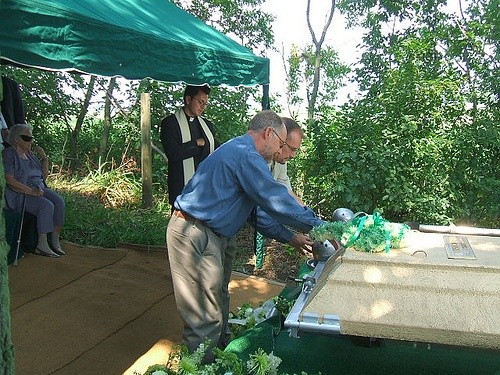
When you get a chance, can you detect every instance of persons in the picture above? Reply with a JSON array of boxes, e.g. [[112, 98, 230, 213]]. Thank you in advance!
[[161, 83, 220, 220], [166, 110, 338, 375], [217, 118, 308, 349], [0, 113, 67, 260]]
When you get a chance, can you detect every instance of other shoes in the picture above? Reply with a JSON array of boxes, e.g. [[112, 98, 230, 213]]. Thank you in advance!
[[35, 248, 59, 257], [51, 245, 65, 255]]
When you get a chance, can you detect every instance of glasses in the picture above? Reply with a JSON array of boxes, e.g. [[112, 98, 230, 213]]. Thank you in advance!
[[286, 142, 298, 153], [19, 134, 33, 141], [273, 130, 286, 148], [193, 97, 210, 107]]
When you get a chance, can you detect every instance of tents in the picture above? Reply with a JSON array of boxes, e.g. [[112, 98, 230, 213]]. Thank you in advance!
[[0, 0, 270, 375]]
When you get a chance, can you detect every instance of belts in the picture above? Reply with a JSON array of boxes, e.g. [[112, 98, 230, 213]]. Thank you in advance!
[[173, 209, 220, 236]]
